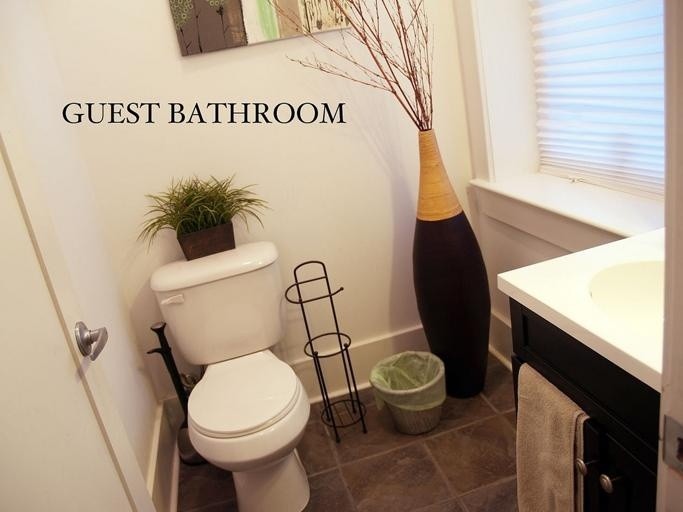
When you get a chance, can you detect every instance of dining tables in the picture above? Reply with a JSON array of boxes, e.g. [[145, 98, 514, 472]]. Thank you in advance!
[[272, 0, 492, 399], [134, 173, 271, 261]]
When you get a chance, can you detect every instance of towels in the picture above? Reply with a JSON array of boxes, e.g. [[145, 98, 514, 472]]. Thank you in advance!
[[369, 351, 445, 435]]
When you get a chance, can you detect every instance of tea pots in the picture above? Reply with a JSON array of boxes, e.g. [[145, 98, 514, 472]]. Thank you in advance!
[[167, 0, 351, 58]]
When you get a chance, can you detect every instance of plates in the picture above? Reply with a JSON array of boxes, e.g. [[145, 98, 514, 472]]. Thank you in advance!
[[498, 226, 664, 510]]
